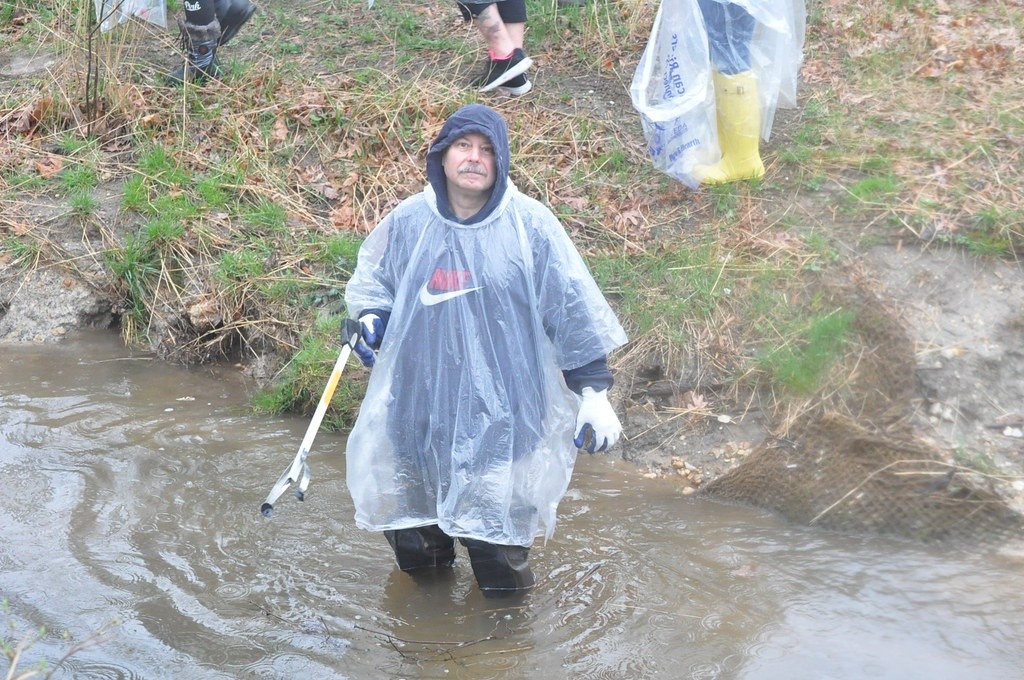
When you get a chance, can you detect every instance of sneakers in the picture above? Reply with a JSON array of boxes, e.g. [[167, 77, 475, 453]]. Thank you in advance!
[[470, 48, 532, 93], [487, 72, 532, 96]]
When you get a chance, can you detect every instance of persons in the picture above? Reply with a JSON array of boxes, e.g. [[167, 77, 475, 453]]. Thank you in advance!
[[691, 0, 766, 186], [456, 0, 534, 96], [350, 104, 623, 594], [162, 0, 257, 87]]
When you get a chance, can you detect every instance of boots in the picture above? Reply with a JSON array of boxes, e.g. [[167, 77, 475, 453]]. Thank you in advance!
[[693, 67, 766, 184], [163, 13, 222, 87], [214, 0, 257, 46]]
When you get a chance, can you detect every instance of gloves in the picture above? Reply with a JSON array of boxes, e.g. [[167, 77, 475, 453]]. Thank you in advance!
[[351, 313, 384, 367], [573, 383, 623, 454]]
